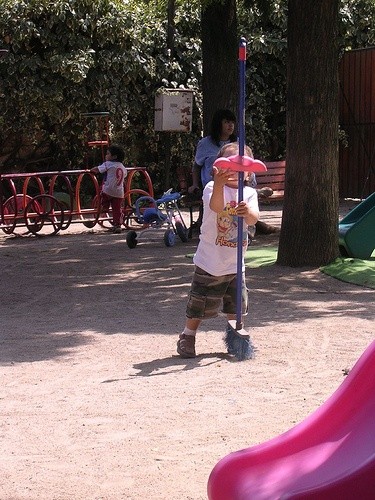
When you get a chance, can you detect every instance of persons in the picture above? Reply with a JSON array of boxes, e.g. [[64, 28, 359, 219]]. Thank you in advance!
[[177, 142, 260, 359], [187, 109, 238, 198], [85, 144, 127, 232]]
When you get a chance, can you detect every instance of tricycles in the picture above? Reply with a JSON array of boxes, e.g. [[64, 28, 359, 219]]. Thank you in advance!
[[126, 193, 189, 248]]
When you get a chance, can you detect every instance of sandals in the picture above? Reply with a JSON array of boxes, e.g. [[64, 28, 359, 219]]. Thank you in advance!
[[228, 341, 258, 353], [177, 333, 196, 358]]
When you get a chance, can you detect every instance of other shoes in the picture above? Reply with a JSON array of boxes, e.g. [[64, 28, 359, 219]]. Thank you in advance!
[[113, 227, 122, 233], [257, 187, 273, 200], [266, 226, 280, 235], [102, 207, 109, 212]]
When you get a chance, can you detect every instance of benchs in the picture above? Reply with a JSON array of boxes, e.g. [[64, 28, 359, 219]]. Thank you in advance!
[[254, 161, 286, 200]]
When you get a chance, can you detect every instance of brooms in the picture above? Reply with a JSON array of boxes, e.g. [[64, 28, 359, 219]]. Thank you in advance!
[[212, 37, 268, 361]]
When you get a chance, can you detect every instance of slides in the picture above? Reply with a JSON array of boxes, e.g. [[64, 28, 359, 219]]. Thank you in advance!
[[207, 337, 375, 500], [338, 190, 375, 259]]
